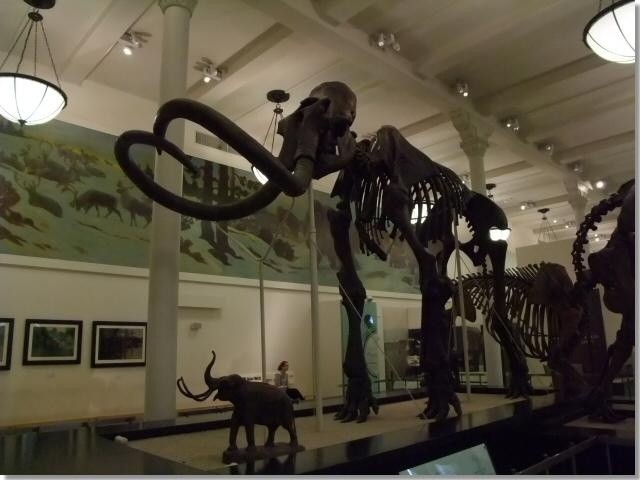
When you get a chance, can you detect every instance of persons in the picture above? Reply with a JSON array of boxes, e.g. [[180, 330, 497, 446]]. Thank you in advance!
[[273, 360, 304, 401]]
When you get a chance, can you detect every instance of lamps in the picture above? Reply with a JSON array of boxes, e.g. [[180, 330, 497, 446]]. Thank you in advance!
[[250, 87, 290, 186], [536, 207, 558, 244], [193, 56, 224, 83], [369, 29, 583, 177], [485, 183, 512, 241], [0, 0, 68, 127], [583, 0, 635, 66], [118, 30, 152, 56]]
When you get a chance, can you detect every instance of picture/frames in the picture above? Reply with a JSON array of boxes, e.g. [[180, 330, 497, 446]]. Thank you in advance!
[[22, 318, 83, 366], [91, 320, 146, 369], [0, 318, 15, 371]]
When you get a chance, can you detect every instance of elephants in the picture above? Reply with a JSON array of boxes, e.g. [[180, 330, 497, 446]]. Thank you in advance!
[[176, 350, 299, 451]]
